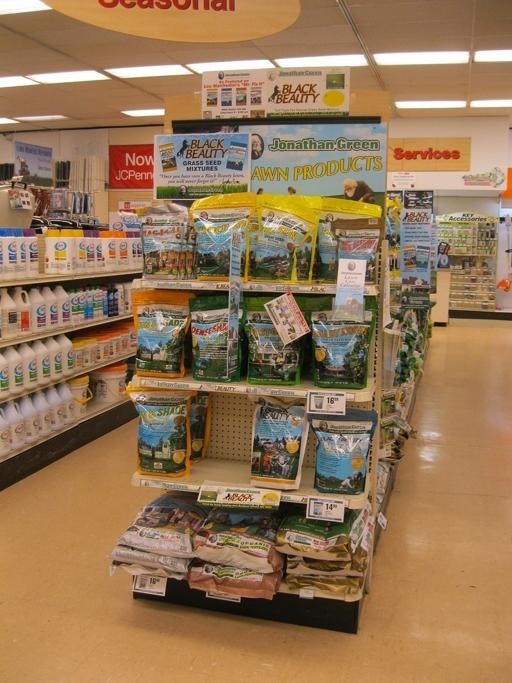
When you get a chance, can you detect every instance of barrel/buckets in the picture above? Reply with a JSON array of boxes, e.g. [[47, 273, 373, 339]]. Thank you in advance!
[[70, 376, 94, 418], [93, 365, 127, 405], [71, 323, 137, 371], [1, 285, 72, 456]]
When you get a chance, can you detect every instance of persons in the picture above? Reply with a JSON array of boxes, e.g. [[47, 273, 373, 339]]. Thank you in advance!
[[343, 179, 374, 203], [251, 136, 262, 159]]
